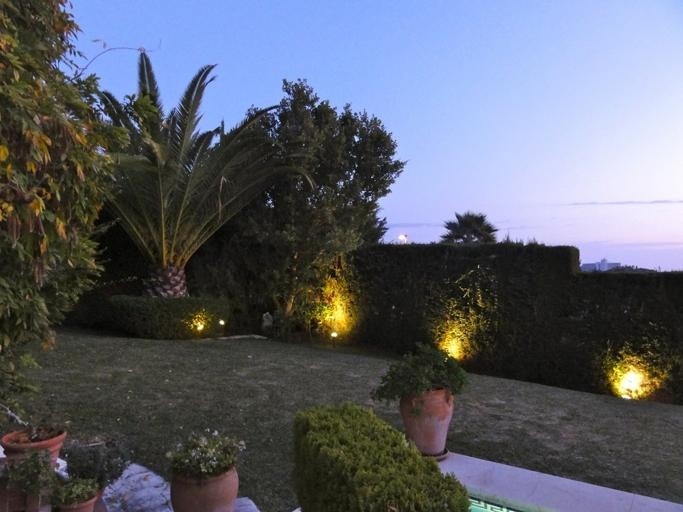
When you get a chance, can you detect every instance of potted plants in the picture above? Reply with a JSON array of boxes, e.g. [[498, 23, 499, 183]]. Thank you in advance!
[[2, 400, 129, 512], [369, 336, 468, 460]]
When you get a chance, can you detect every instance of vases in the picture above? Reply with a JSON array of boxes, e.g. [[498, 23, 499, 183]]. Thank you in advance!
[[170, 469, 241, 512]]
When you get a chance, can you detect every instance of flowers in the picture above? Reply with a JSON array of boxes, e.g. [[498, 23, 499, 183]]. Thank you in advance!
[[161, 424, 249, 483]]
